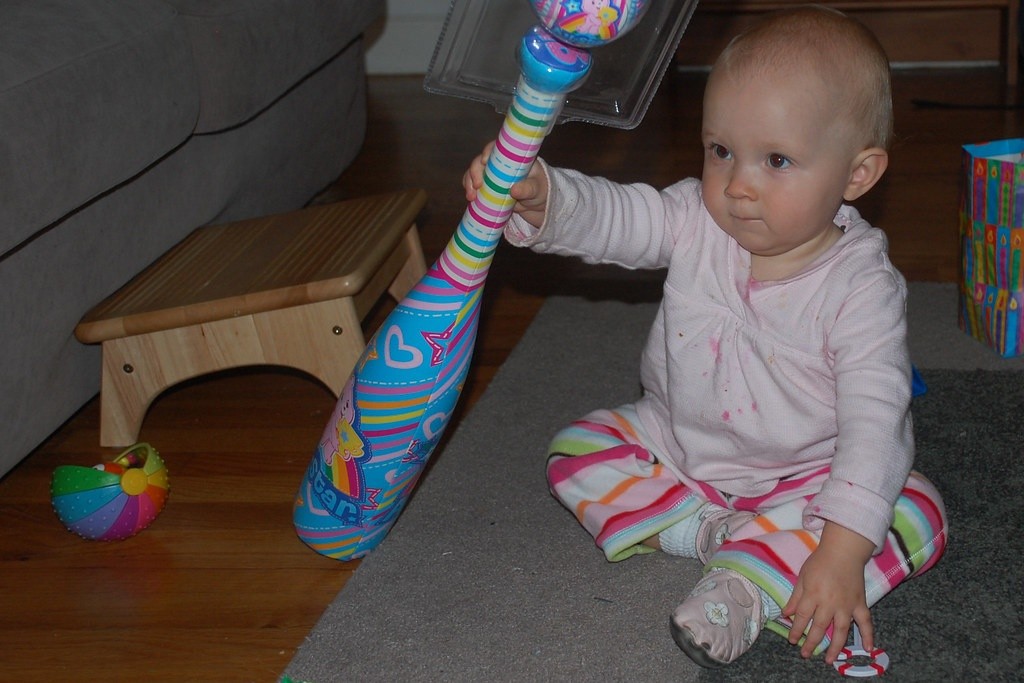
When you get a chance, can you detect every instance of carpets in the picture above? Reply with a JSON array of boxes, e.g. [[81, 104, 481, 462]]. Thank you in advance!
[[280, 280, 1024, 683]]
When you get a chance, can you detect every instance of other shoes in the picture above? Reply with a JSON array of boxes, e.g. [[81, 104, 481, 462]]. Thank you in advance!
[[669, 569, 763, 669], [694, 510, 759, 566]]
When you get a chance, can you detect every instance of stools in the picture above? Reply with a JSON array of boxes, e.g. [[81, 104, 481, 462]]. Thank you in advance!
[[74, 186, 429, 447]]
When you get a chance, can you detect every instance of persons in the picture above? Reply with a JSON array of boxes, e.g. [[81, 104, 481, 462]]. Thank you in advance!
[[461, 6, 949, 669]]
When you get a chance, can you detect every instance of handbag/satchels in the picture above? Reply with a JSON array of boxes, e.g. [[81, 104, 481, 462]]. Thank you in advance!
[[958, 138, 1024, 357]]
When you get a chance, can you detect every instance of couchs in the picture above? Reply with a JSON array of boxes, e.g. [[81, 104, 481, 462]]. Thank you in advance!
[[0, 0, 386, 482]]
